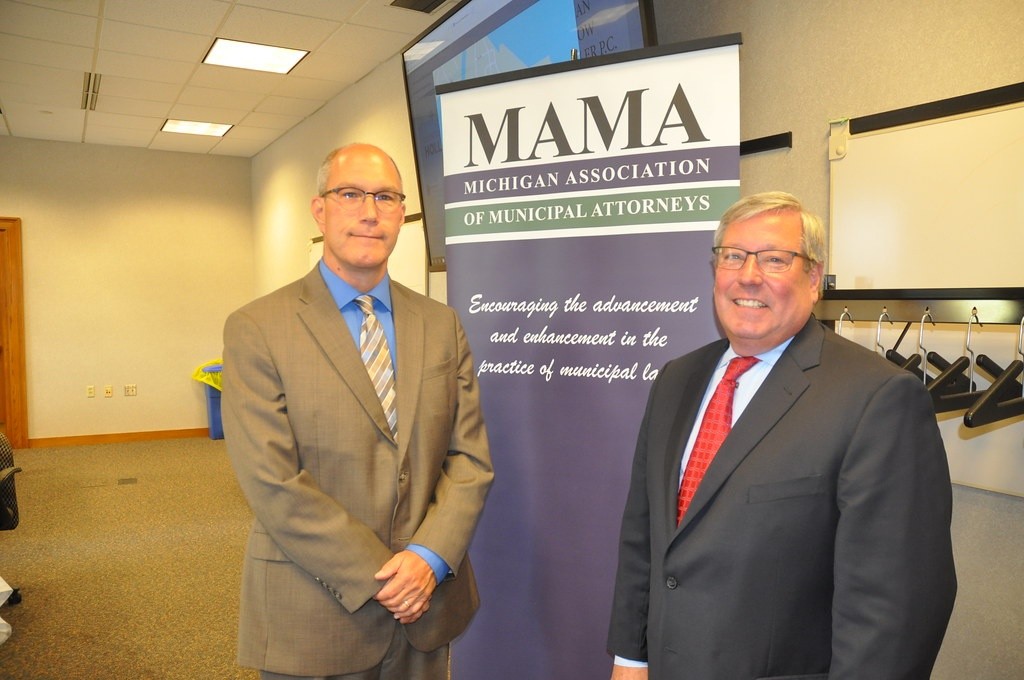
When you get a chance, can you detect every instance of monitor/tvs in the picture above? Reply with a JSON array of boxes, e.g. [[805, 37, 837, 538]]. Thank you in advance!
[[400, 0, 658, 272]]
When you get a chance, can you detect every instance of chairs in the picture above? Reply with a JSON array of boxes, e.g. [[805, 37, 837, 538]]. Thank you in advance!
[[0, 432, 23, 604]]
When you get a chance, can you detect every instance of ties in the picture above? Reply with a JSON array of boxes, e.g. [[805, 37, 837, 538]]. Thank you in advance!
[[353, 294, 399, 446], [676, 356, 760, 527]]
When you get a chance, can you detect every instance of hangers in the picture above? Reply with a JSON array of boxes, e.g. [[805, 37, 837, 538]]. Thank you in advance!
[[835, 310, 1024, 427]]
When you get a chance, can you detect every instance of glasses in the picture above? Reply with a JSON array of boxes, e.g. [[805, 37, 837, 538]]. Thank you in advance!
[[321, 187, 406, 214], [711, 246, 814, 273]]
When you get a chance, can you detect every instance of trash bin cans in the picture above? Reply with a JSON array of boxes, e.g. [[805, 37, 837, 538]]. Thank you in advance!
[[203, 365, 225, 440]]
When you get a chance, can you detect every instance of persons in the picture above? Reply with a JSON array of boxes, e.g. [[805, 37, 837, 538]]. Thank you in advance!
[[221, 143, 495, 680], [606, 191, 958, 680]]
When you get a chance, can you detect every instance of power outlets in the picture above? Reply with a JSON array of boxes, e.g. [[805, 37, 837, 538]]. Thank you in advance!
[[104, 385, 112, 397]]
